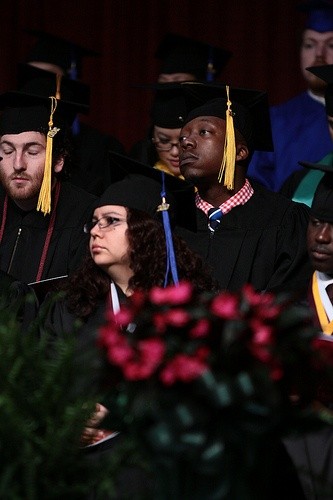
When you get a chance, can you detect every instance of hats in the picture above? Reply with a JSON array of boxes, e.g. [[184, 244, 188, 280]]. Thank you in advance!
[[0, 90, 91, 216], [291, 0, 333, 33], [14, 61, 90, 105], [153, 32, 232, 82], [128, 83, 196, 129], [173, 77, 274, 191], [307, 64, 333, 117], [72, 150, 194, 288], [296, 160, 333, 224], [23, 28, 101, 80]]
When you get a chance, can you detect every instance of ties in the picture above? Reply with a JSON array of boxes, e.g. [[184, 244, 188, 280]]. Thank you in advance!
[[208, 208, 222, 237]]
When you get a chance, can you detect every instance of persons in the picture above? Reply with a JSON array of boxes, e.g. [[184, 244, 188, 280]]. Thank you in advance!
[[1, 0, 333, 442]]
